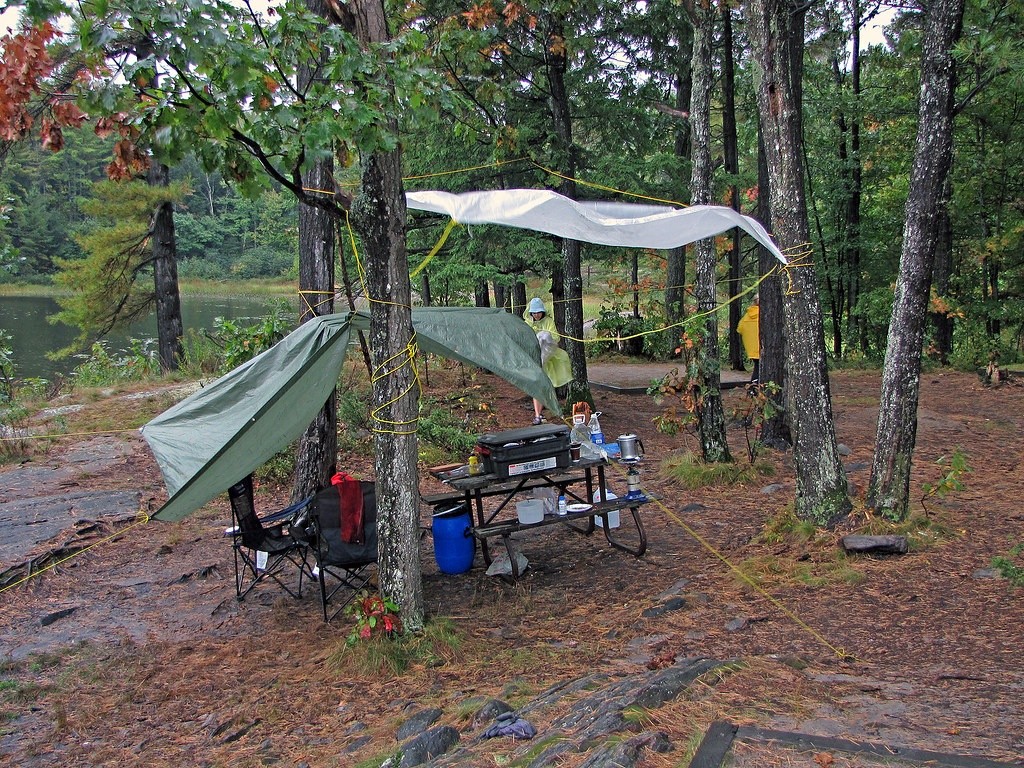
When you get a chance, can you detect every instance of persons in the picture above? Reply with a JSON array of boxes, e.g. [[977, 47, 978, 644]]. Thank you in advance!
[[524, 298, 573, 425], [737, 293, 759, 393]]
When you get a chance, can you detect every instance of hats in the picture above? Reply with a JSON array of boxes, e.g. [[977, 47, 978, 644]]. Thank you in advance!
[[751, 293, 759, 301], [528, 298, 546, 313]]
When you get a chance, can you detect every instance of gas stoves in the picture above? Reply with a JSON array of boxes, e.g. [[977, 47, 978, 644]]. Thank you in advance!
[[618, 458, 648, 465]]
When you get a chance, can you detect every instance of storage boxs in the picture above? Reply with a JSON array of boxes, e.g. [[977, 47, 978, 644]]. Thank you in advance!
[[477, 423, 571, 481]]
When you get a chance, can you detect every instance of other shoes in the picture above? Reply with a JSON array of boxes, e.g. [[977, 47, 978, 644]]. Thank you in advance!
[[533, 414, 548, 424], [747, 390, 758, 398]]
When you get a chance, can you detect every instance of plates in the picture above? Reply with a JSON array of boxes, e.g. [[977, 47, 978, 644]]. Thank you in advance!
[[566, 504, 593, 512]]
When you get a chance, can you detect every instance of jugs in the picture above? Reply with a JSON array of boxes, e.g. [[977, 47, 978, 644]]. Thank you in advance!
[[533, 487, 557, 515]]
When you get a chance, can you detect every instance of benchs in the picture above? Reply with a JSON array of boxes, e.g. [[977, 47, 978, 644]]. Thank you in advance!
[[470, 492, 663, 577], [422, 474, 599, 530]]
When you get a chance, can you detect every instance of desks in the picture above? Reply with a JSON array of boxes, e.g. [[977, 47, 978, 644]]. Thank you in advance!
[[450, 457, 640, 580]]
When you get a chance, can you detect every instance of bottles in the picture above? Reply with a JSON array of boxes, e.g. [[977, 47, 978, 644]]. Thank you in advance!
[[587, 411, 605, 448], [594, 486, 620, 529], [468, 456, 480, 474], [569, 414, 591, 442], [558, 496, 567, 516]]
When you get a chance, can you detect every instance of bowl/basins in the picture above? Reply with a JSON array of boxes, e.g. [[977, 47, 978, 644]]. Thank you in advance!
[[515, 499, 544, 524], [224, 525, 240, 536]]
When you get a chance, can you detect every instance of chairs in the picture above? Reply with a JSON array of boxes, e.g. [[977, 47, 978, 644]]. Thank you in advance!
[[228, 469, 318, 600], [288, 479, 377, 623]]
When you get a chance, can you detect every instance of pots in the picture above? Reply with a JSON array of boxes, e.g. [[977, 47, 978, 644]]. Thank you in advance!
[[615, 433, 645, 455]]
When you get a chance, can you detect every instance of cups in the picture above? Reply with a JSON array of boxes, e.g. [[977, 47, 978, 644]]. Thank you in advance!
[[569, 442, 581, 463]]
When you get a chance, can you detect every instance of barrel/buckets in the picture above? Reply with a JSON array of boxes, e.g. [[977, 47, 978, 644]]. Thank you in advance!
[[430, 500, 475, 575]]
